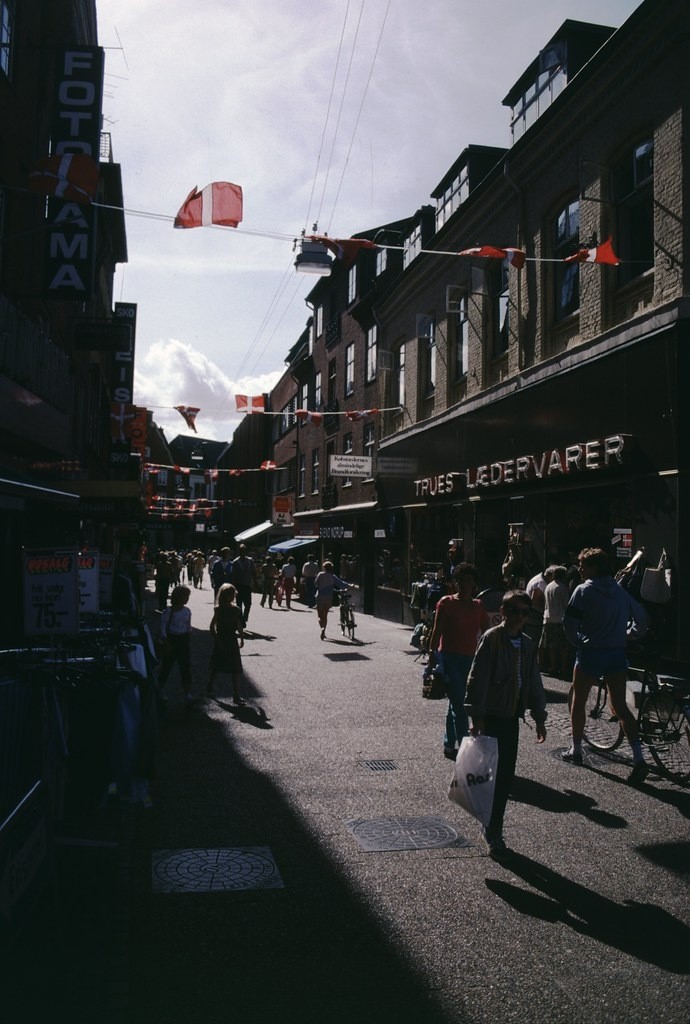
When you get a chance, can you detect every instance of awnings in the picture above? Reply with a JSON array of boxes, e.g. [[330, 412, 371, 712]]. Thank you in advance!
[[234, 520, 294, 547], [293, 501, 378, 521], [269, 536, 320, 554]]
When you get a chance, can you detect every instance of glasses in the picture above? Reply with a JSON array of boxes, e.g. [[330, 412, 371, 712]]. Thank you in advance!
[[507, 606, 530, 616]]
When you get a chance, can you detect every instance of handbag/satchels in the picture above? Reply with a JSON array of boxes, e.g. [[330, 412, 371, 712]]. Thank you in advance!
[[423, 669, 449, 699], [447, 734, 499, 829], [640, 554, 674, 605]]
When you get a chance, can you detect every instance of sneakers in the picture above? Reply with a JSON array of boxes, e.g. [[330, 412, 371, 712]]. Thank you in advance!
[[483, 828, 508, 859], [627, 759, 648, 785], [561, 750, 584, 765]]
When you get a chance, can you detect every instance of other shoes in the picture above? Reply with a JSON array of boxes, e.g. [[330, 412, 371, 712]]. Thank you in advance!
[[321, 627, 326, 639], [233, 693, 246, 707], [444, 741, 455, 758], [206, 678, 214, 693]]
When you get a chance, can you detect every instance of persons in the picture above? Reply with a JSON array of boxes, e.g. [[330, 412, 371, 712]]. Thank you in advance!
[[142, 544, 594, 760], [560, 547, 649, 785], [463, 589, 548, 861]]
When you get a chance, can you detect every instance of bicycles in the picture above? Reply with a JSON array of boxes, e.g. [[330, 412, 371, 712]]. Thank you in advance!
[[567, 629, 690, 782], [333, 587, 355, 641]]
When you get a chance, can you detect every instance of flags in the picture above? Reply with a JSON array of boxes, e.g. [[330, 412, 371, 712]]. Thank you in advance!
[[344, 409, 379, 423], [294, 411, 322, 427], [109, 403, 133, 438], [461, 246, 526, 269], [174, 182, 242, 230], [305, 236, 377, 262], [261, 460, 277, 472], [235, 395, 264, 414], [131, 408, 146, 448], [565, 234, 620, 266], [141, 462, 243, 517], [173, 406, 200, 433]]
